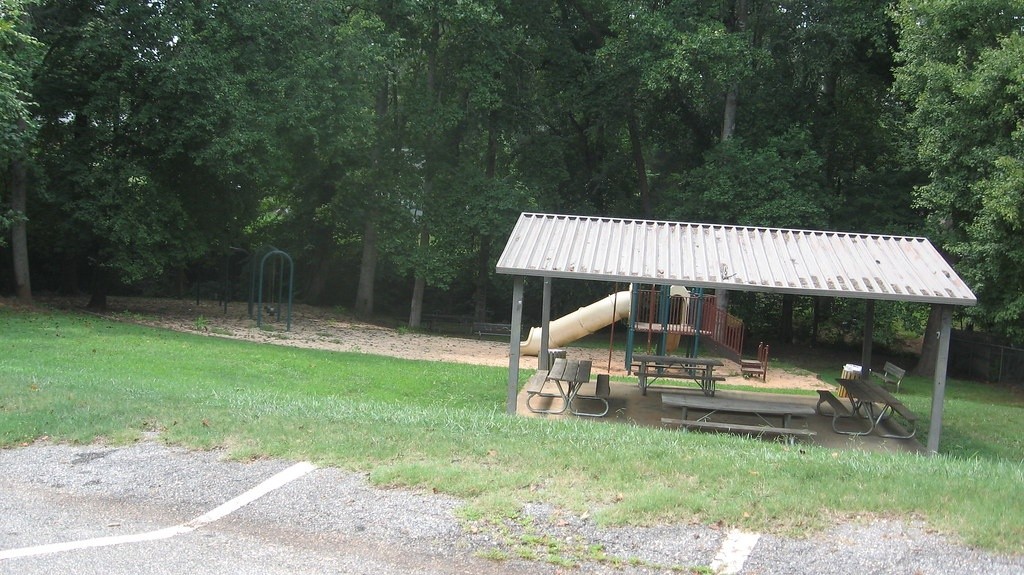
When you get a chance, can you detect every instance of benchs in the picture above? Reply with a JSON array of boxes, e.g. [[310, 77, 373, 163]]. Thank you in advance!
[[873, 362, 907, 395], [662, 416, 817, 443], [817, 389, 854, 435], [628, 363, 727, 394], [525, 368, 550, 412], [889, 402, 918, 439], [592, 374, 611, 415]]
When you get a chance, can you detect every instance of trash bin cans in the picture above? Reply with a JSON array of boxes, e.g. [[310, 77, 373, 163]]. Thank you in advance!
[[837, 363, 871, 399]]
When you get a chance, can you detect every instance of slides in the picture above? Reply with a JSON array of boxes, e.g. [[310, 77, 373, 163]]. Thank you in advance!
[[520, 290, 628, 355]]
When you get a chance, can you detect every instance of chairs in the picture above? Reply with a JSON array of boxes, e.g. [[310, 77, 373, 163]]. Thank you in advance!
[[742, 345, 771, 382], [741, 342, 766, 371]]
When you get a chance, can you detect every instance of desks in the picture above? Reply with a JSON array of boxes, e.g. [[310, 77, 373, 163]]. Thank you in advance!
[[547, 357, 593, 417], [660, 392, 815, 429], [632, 354, 724, 396], [836, 376, 901, 438]]
